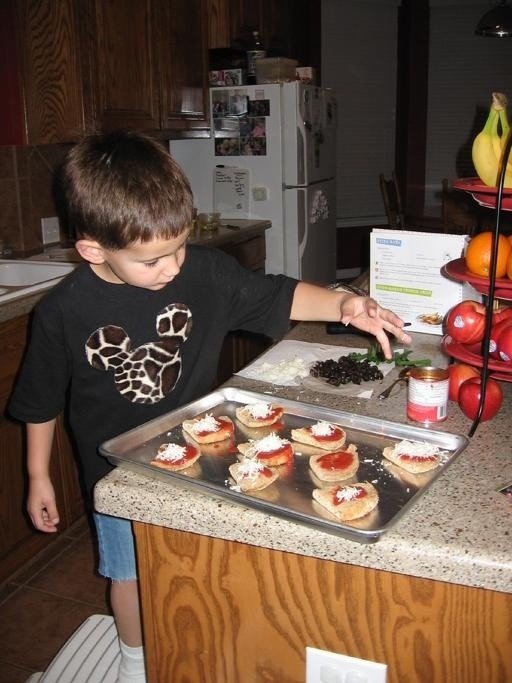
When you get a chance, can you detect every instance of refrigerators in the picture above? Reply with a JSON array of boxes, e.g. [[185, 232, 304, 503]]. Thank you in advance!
[[209, 80, 338, 287]]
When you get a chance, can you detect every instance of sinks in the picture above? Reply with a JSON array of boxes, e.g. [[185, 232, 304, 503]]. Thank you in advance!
[[0, 259, 80, 304]]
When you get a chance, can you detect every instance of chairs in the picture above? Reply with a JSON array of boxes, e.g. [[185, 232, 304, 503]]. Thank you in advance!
[[438, 174, 480, 237], [379, 170, 408, 230]]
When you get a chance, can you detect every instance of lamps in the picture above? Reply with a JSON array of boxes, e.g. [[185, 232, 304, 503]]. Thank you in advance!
[[473, 0, 512, 38]]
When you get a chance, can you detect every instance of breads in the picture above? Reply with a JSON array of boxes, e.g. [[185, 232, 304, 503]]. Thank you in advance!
[[312, 482, 378, 520], [152, 443, 200, 471], [383, 439, 439, 473], [310, 444, 359, 482], [236, 402, 283, 428], [181, 414, 234, 443], [229, 463, 279, 492], [291, 421, 345, 450], [237, 434, 294, 466]]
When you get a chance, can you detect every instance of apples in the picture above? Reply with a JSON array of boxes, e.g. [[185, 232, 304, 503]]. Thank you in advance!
[[447, 299, 491, 344], [487, 317, 511, 364], [497, 326, 512, 361], [459, 377, 503, 423], [492, 307, 512, 324], [446, 364, 482, 402]]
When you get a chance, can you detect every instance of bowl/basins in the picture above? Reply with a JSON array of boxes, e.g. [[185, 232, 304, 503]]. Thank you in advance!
[[199, 213, 221, 230]]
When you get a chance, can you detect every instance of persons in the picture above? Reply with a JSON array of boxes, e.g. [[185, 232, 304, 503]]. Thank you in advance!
[[7, 129, 414, 682]]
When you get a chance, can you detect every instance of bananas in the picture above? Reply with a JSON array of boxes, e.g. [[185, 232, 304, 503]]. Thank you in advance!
[[471, 93, 512, 187]]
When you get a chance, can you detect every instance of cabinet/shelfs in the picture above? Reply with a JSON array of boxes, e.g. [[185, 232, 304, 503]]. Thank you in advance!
[[211, 230, 267, 385], [0, 312, 86, 593]]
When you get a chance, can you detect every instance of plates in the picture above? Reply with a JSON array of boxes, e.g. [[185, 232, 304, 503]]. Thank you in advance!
[[440, 258, 512, 301], [441, 333, 512, 382], [451, 177, 512, 212]]
